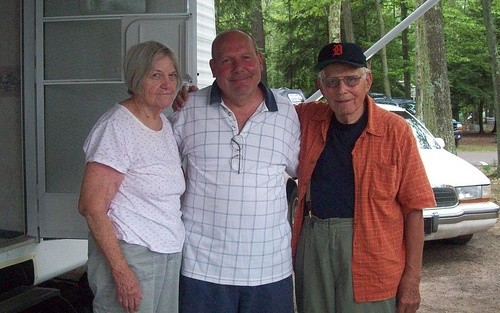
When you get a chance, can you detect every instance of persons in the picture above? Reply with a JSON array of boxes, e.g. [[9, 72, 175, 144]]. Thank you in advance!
[[173, 43, 437, 313], [167, 29, 301, 313], [78, 41, 186, 313]]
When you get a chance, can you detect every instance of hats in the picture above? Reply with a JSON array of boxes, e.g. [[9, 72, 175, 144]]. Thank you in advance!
[[314, 43, 367, 71]]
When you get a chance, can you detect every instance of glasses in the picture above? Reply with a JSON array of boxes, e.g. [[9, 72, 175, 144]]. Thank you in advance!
[[319, 72, 366, 88], [230, 134, 244, 174]]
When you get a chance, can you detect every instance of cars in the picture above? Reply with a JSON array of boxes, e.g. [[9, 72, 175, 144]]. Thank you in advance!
[[372, 98, 464, 147], [376, 103, 500, 243], [279, 88, 307, 105]]
